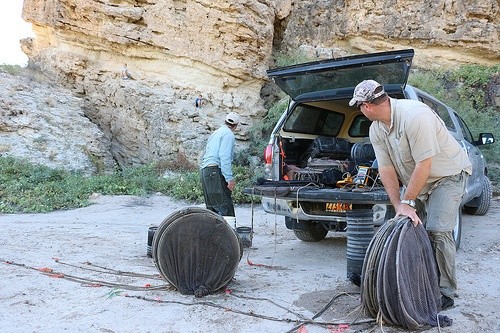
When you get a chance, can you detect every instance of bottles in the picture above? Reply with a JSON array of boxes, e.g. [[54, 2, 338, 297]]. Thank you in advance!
[[236, 226, 252, 248], [147, 227, 159, 257]]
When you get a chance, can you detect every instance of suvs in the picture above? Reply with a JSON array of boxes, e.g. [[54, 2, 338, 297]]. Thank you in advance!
[[243, 49, 494, 254]]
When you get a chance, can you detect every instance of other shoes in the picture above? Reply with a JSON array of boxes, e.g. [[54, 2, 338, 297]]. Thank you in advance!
[[441, 295, 454, 310]]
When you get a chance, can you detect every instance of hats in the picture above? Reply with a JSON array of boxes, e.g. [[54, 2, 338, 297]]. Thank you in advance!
[[348, 79, 385, 106], [225, 112, 239, 125]]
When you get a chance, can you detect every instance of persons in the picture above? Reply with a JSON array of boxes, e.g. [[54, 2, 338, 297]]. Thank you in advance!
[[201, 112, 240, 229], [349, 79, 473, 310]]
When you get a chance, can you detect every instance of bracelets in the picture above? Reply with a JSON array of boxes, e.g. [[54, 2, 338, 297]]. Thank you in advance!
[[401, 199, 416, 208]]
